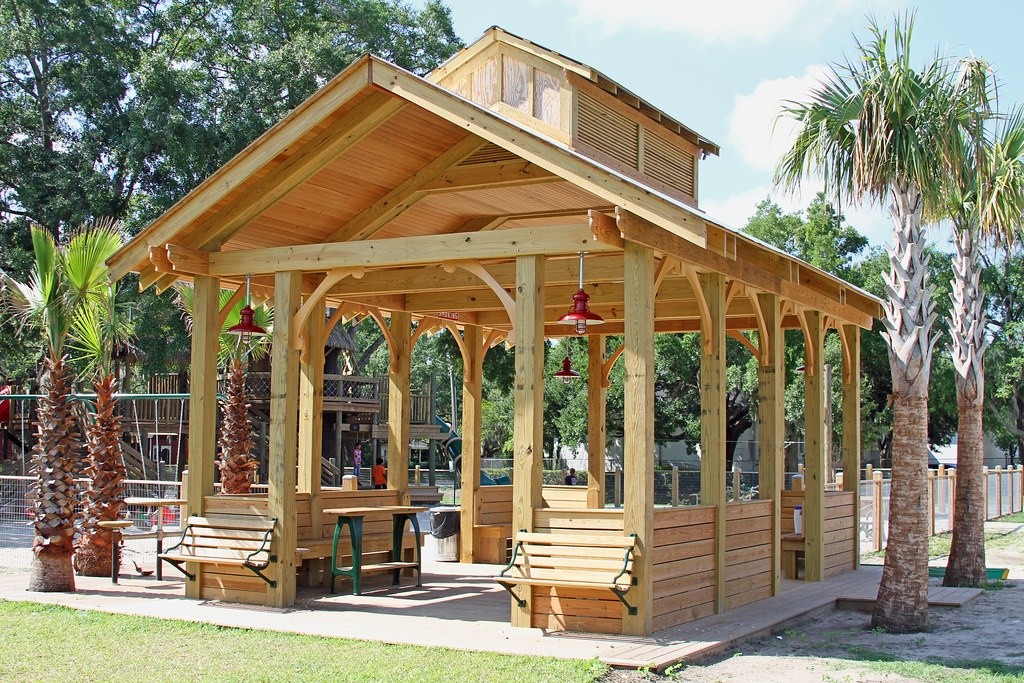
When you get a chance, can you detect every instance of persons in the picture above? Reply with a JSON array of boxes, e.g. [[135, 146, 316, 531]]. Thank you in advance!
[[372, 458, 387, 489], [353, 443, 361, 478], [151, 506, 173, 526], [565, 468, 577, 485]]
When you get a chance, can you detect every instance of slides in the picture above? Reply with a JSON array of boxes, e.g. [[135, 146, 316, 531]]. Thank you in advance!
[[436, 416, 512, 486]]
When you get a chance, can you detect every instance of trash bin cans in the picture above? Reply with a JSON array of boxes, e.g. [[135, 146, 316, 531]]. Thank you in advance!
[[429, 506, 460, 562]]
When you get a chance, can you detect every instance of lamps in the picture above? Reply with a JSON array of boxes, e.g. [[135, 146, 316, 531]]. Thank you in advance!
[[225, 274, 268, 339], [552, 336, 580, 385], [554, 250, 606, 335]]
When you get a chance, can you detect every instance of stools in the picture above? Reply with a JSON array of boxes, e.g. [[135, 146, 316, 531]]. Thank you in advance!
[[98, 521, 133, 584]]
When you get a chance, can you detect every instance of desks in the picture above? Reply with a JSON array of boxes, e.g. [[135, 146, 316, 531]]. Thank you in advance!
[[322, 505, 430, 597], [124, 497, 187, 580]]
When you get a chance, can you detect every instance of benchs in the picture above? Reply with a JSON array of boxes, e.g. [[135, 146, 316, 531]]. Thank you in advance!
[[496, 529, 638, 618], [473, 485, 588, 565], [297, 491, 430, 589], [159, 515, 278, 590], [780, 490, 856, 580]]
